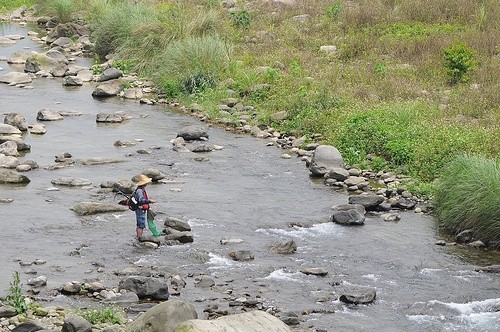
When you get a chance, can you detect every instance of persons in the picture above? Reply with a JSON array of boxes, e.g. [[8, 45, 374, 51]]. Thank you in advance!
[[130, 174, 157, 238]]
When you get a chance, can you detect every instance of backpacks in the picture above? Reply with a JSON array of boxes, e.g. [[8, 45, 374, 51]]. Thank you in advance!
[[129, 196, 138, 211]]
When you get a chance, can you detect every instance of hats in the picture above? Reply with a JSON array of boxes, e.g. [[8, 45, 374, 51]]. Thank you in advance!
[[136, 174, 153, 186]]
